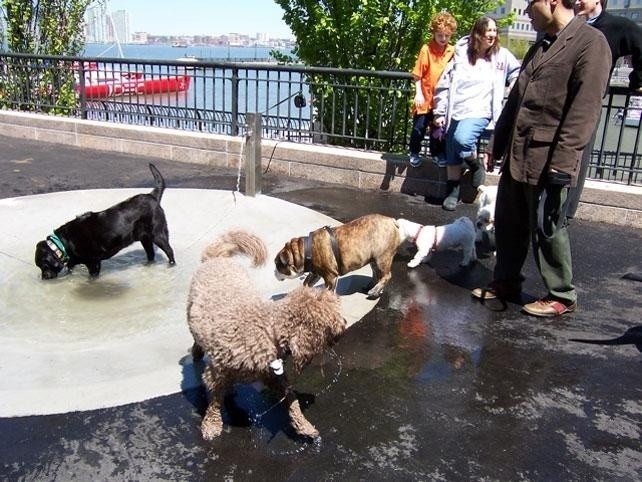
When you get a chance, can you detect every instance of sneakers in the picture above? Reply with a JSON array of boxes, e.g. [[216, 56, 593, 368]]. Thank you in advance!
[[432, 155, 447, 167], [473, 283, 522, 299], [409, 151, 421, 167], [523, 297, 578, 318]]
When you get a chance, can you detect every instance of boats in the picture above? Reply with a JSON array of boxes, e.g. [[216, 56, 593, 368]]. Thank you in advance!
[[612, 96, 642, 127]]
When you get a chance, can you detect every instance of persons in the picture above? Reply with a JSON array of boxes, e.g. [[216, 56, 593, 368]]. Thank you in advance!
[[470, 1, 616, 318], [561, 1, 642, 229], [408, 10, 457, 170], [432, 14, 521, 211]]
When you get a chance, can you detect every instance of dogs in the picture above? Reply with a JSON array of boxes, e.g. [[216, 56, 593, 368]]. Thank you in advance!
[[396, 216, 478, 268], [475, 185, 498, 257], [34, 163, 177, 284], [274, 213, 400, 298], [186, 229, 347, 442]]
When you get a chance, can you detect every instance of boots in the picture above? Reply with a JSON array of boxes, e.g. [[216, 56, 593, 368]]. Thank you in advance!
[[467, 156, 486, 187], [444, 179, 460, 209]]
